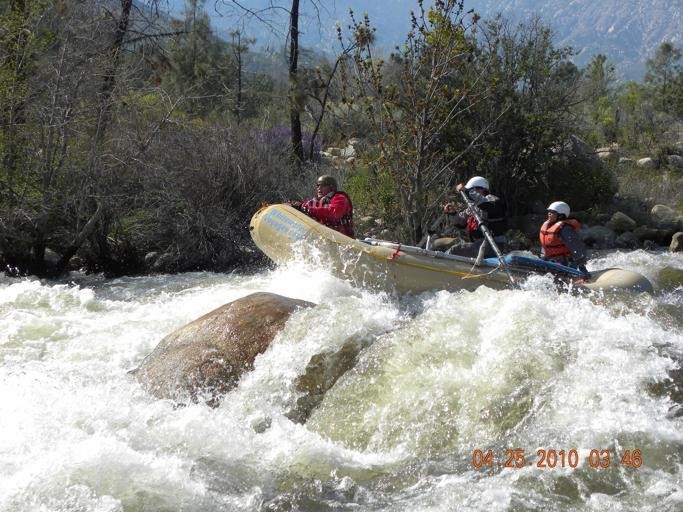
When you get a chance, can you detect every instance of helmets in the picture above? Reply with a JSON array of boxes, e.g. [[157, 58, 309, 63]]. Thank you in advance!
[[546, 201, 571, 219], [317, 175, 337, 186], [464, 176, 490, 192]]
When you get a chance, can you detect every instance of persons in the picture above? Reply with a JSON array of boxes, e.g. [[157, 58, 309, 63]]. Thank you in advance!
[[443, 175, 507, 259], [287, 174, 356, 242], [538, 200, 592, 284]]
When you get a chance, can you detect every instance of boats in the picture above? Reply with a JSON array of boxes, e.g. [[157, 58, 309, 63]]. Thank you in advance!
[[246, 202, 653, 311]]
